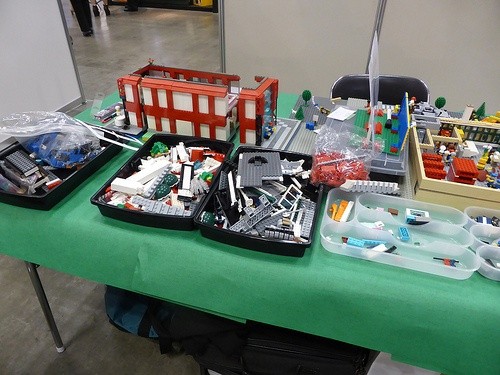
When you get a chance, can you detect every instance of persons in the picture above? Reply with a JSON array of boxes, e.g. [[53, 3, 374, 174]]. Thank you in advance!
[[70, 0, 94, 37], [92, 0, 138, 17]]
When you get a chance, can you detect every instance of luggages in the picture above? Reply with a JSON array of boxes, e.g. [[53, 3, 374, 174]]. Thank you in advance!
[[240, 319, 369, 375]]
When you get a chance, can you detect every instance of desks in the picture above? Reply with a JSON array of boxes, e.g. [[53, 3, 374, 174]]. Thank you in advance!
[[0, 87, 500, 375]]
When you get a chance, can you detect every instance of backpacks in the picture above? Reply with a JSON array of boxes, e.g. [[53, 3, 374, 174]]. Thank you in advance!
[[104, 285, 245, 375]]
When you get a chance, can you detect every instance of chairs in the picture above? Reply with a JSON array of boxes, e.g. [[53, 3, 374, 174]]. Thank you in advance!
[[329, 74, 430, 108]]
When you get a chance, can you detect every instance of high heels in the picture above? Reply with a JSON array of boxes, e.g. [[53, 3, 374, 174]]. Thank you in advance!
[[93, 5, 100, 17], [104, 5, 110, 16]]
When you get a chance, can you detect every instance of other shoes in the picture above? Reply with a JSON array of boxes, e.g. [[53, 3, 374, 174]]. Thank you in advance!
[[124, 5, 138, 11]]
